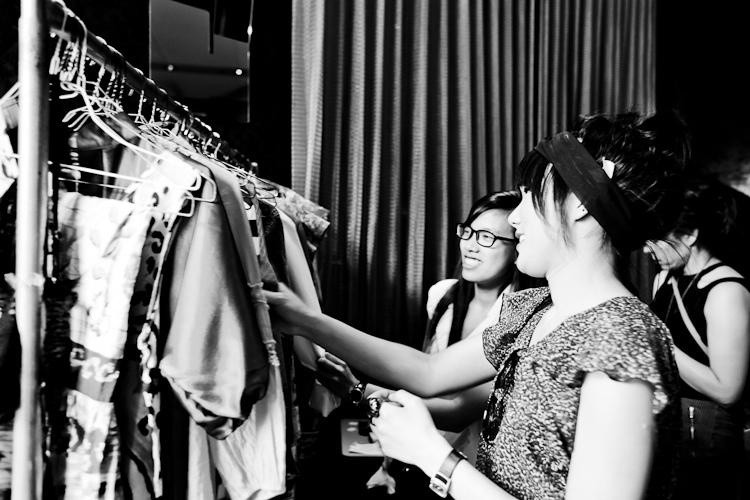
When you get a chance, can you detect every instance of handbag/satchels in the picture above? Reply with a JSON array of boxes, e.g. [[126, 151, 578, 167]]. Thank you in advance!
[[666, 276, 748, 460]]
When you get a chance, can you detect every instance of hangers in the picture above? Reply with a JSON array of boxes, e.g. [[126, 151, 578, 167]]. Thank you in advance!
[[1, 1, 281, 223]]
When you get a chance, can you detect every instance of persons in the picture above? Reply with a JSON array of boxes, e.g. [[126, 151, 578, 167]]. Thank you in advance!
[[641, 181, 750, 498], [316, 192, 536, 500], [262, 110, 718, 497]]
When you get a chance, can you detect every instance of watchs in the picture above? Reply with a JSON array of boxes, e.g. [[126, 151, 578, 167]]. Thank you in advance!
[[347, 374, 371, 404], [428, 451, 469, 498]]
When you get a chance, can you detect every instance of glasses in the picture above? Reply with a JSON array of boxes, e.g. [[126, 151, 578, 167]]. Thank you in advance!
[[454, 221, 519, 249]]
